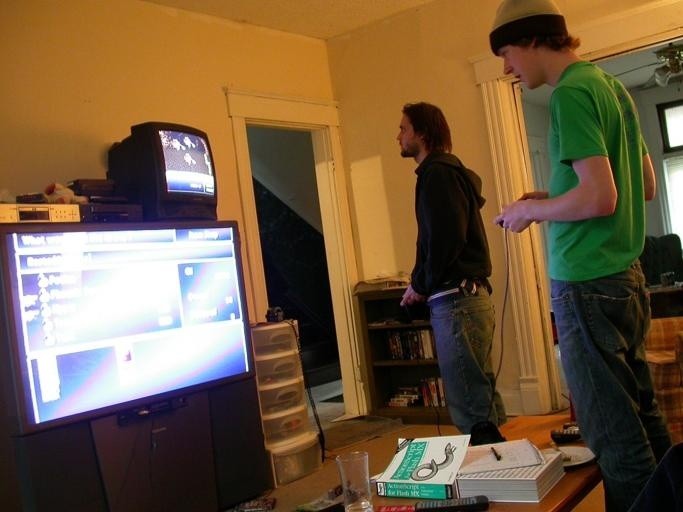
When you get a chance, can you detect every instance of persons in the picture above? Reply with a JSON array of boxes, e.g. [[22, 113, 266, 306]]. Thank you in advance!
[[396, 102, 506, 443], [489, 0, 672, 512]]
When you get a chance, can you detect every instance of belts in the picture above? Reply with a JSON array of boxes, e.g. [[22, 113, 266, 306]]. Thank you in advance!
[[426, 278, 479, 306]]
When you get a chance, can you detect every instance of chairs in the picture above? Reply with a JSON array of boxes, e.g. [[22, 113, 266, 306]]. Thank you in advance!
[[640, 317, 682, 446]]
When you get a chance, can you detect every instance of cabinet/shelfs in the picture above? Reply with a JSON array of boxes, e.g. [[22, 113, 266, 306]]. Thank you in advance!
[[250, 318, 322, 487], [8, 379, 277, 510], [356, 282, 456, 427]]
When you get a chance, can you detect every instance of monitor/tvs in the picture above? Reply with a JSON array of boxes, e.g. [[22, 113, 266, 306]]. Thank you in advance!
[[105, 119, 220, 221], [1, 219, 257, 438]]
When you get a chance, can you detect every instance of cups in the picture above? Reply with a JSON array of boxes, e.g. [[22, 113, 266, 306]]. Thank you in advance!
[[335, 450, 375, 512], [659, 271, 675, 286]]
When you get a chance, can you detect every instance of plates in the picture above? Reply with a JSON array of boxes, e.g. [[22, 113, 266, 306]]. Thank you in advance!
[[539, 445, 596, 467]]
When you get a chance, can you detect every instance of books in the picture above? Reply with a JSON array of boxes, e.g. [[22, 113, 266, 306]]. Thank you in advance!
[[381, 329, 446, 410], [375, 433, 472, 500]]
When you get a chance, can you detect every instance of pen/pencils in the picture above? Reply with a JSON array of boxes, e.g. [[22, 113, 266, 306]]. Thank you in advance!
[[491, 447, 501, 461]]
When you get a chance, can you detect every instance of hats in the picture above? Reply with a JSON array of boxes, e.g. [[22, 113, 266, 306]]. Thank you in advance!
[[487, 1, 566, 37]]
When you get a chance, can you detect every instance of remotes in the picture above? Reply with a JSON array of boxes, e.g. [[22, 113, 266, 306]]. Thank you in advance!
[[414, 496, 490, 512], [550, 429, 581, 444]]
